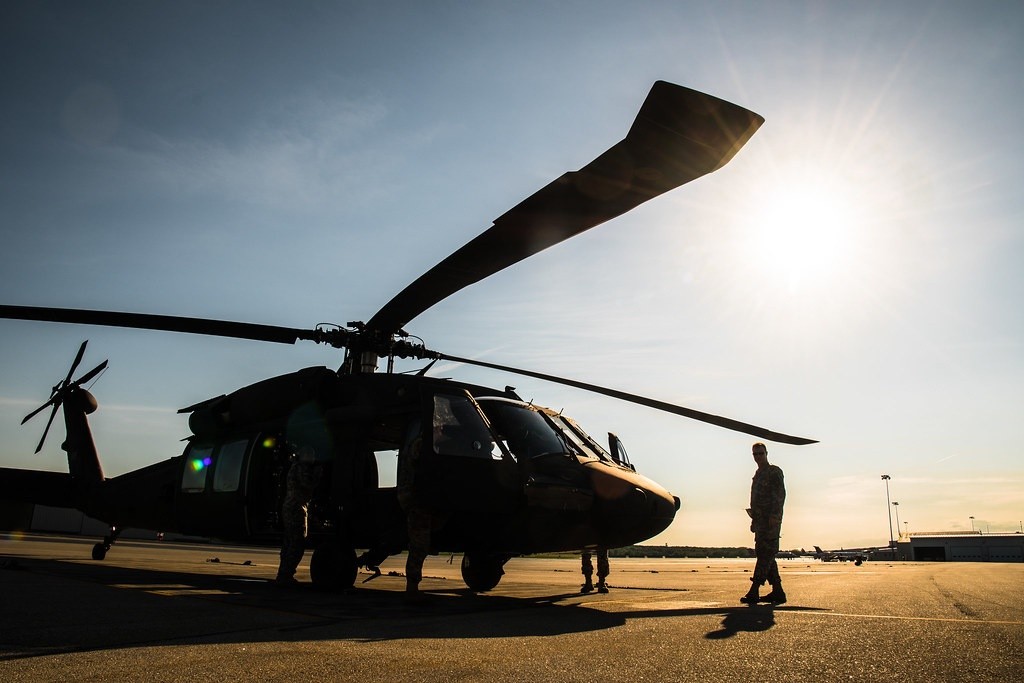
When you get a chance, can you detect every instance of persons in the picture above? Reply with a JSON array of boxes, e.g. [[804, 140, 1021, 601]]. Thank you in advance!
[[581, 549, 609, 593], [740, 443, 785, 603]]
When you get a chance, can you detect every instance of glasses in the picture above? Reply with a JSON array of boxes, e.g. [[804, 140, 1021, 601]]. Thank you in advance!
[[753, 452, 765, 455]]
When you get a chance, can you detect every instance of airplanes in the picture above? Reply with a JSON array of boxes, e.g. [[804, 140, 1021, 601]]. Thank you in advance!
[[774, 552, 797, 560], [800, 546, 863, 560]]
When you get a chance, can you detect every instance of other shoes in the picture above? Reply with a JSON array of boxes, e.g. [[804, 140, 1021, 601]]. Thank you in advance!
[[581, 582, 594, 593], [598, 585, 609, 593], [740, 591, 760, 603], [760, 591, 787, 604]]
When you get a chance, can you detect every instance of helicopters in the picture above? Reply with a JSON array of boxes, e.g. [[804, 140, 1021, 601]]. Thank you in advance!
[[0, 80, 820, 599]]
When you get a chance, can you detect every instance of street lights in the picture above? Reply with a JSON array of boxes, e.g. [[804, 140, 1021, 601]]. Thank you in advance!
[[881, 472, 896, 561]]
